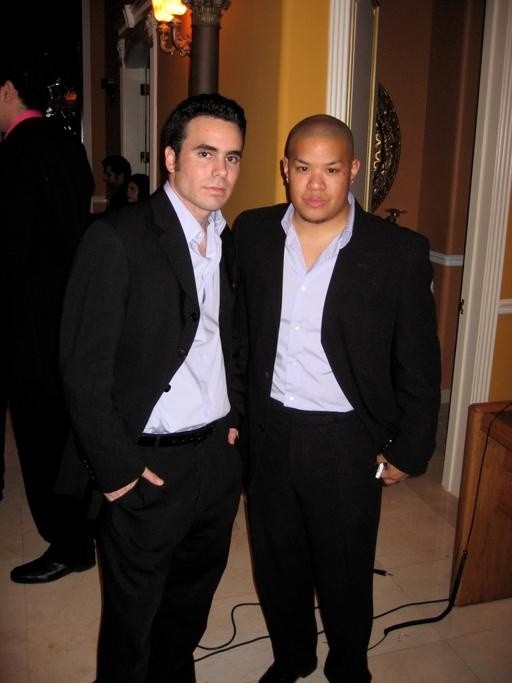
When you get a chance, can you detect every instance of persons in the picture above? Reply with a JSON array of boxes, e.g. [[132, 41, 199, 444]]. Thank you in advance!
[[53, 88, 249, 680], [93, 152, 133, 219], [217, 108, 447, 682], [1, 50, 98, 587], [124, 171, 152, 205], [28, 51, 83, 140]]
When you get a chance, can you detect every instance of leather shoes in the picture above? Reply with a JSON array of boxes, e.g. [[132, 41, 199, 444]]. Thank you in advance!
[[255, 654, 318, 682], [8, 552, 98, 584]]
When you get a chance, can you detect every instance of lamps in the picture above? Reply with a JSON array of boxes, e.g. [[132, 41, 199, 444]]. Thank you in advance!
[[151, 0, 192, 55]]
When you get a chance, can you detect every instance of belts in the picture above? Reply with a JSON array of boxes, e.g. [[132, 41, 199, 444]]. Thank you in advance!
[[134, 418, 217, 449]]
